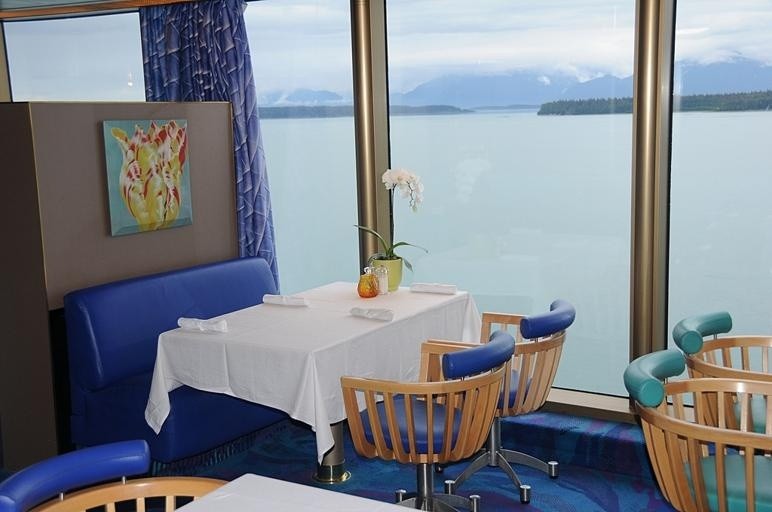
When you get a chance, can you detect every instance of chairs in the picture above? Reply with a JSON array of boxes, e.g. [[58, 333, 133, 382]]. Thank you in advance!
[[428, 298, 576, 503], [339, 328, 515, 512], [0, 440, 230, 511], [624, 311, 772, 512]]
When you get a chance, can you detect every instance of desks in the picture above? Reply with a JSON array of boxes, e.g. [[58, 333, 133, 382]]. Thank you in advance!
[[174, 471, 425, 512], [155, 280, 469, 484]]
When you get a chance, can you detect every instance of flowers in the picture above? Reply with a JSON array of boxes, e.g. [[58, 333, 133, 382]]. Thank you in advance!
[[353, 168, 429, 273]]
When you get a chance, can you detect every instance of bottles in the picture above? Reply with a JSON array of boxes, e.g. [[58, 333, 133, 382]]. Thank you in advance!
[[357, 266, 379, 298], [375, 263, 389, 296]]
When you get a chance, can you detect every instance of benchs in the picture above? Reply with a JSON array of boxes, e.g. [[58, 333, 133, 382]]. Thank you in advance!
[[63, 256, 290, 472]]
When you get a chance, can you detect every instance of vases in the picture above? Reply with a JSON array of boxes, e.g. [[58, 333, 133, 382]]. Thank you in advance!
[[369, 258, 403, 292]]
